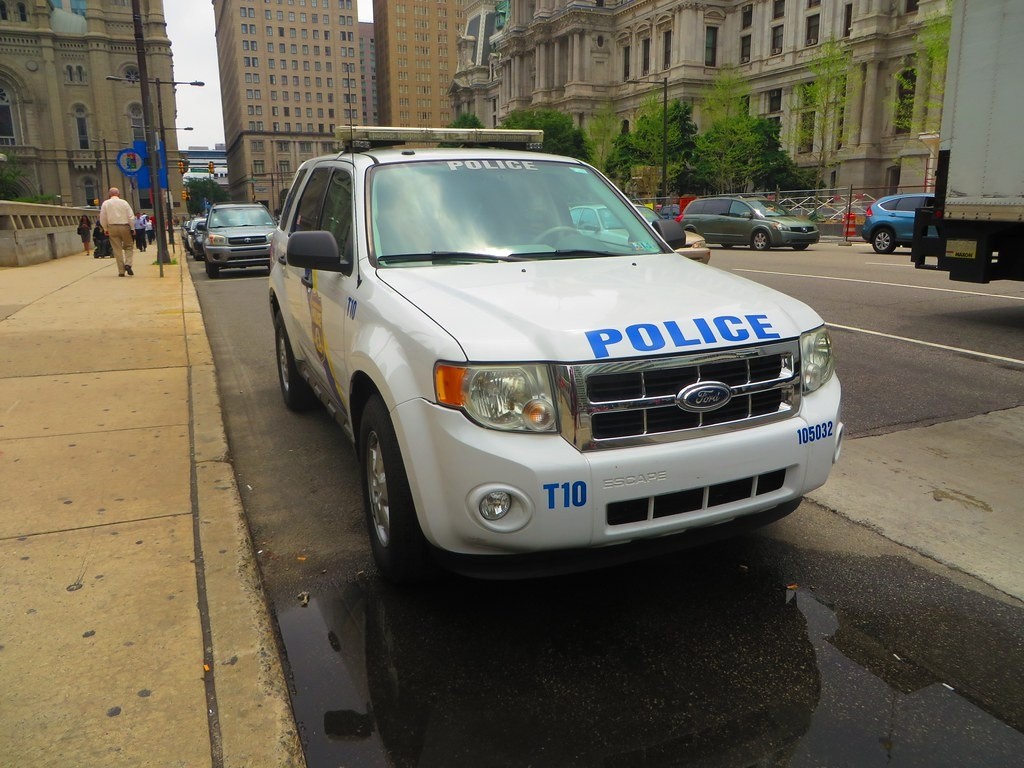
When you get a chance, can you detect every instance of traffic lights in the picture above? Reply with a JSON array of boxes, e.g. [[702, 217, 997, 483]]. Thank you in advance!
[[177, 162, 184, 174], [182, 191, 187, 200]]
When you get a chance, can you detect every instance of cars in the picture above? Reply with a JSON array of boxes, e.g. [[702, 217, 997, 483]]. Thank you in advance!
[[569, 204, 711, 266], [661, 194, 820, 251], [181, 217, 207, 261], [861, 194, 939, 255]]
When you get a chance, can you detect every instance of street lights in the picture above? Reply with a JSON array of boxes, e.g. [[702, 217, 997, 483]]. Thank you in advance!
[[106, 76, 205, 245], [626, 77, 668, 206]]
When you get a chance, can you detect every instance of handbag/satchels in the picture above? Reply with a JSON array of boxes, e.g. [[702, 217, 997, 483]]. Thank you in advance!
[[77, 228, 82, 234]]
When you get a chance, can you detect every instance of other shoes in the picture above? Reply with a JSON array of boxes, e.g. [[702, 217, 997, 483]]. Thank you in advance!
[[119, 274, 124, 276], [126, 265, 134, 275]]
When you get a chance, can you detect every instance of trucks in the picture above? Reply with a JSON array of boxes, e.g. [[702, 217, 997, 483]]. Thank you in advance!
[[909, 0, 1024, 284]]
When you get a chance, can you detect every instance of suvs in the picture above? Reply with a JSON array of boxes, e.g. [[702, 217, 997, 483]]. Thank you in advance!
[[267, 126, 845, 559], [198, 201, 282, 278]]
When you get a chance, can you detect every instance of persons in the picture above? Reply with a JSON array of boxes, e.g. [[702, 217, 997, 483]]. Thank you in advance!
[[79, 215, 91, 256], [100, 187, 136, 277], [93, 214, 114, 258], [131, 212, 156, 252]]
